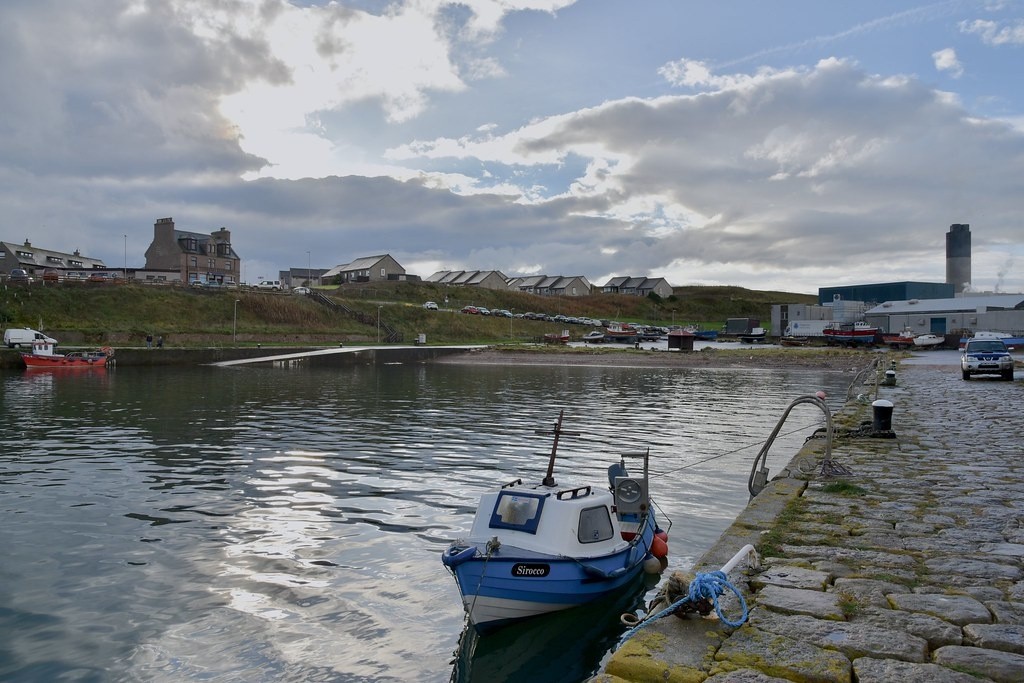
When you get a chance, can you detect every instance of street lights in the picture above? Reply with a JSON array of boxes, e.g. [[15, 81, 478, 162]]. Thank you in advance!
[[307, 251, 310, 290], [378, 306, 383, 343], [124, 235, 126, 281], [234, 299, 240, 342]]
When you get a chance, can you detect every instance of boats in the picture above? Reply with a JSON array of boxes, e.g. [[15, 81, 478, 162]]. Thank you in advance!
[[544, 330, 570, 343], [20, 340, 116, 367], [442, 409, 673, 638], [823, 322, 946, 347], [738, 327, 768, 343], [583, 321, 668, 342]]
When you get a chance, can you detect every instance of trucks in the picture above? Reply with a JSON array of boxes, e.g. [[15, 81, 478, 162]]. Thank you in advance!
[[784, 320, 840, 337]]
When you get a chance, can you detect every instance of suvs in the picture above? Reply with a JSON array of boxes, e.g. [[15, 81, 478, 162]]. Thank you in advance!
[[250, 280, 283, 292], [958, 337, 1015, 380]]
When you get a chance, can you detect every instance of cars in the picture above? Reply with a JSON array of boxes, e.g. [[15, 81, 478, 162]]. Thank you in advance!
[[193, 280, 221, 288], [151, 278, 164, 285], [65, 271, 81, 279], [88, 272, 106, 281], [223, 281, 238, 289], [294, 286, 309, 295], [461, 306, 610, 327], [423, 302, 438, 310], [42, 268, 58, 282], [10, 269, 28, 282]]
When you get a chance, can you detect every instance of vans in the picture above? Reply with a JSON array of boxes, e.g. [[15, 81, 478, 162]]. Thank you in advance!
[[3, 327, 58, 349]]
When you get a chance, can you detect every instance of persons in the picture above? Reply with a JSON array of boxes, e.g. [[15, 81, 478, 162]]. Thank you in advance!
[[157, 336, 163, 347], [146, 334, 152, 349]]
